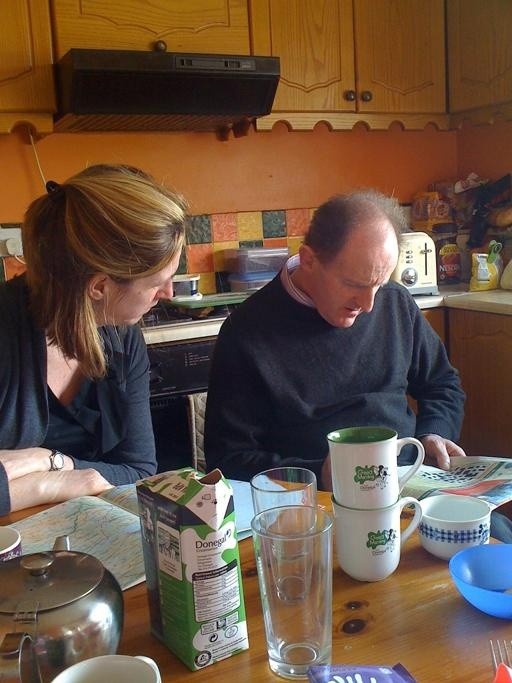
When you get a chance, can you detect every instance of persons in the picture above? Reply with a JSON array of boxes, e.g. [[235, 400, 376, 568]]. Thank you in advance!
[[201, 186, 511, 546], [1, 162, 190, 518]]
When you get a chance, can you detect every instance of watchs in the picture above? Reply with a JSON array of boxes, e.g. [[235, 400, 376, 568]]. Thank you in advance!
[[48, 448, 65, 471]]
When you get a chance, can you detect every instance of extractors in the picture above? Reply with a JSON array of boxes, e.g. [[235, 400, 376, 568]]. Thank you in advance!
[[51, 48, 281, 142]]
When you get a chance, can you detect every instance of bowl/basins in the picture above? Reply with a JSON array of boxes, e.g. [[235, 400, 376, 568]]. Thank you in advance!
[[448, 543, 512, 621]]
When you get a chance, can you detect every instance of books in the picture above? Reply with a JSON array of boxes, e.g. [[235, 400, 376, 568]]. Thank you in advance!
[[6, 477, 285, 591], [393, 454, 511, 512]]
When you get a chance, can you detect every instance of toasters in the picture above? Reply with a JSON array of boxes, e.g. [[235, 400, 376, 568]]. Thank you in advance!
[[388, 232, 440, 297]]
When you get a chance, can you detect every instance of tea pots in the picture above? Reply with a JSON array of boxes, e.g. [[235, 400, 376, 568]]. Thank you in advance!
[[0, 534, 125, 683]]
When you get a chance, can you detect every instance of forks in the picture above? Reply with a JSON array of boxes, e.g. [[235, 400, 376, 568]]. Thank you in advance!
[[490, 640, 512, 678]]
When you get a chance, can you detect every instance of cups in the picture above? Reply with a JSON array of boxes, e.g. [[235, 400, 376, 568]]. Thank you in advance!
[[326, 425, 426, 583], [249, 466, 335, 682], [0, 526, 24, 561], [416, 494, 492, 562], [50, 654, 162, 683]]
